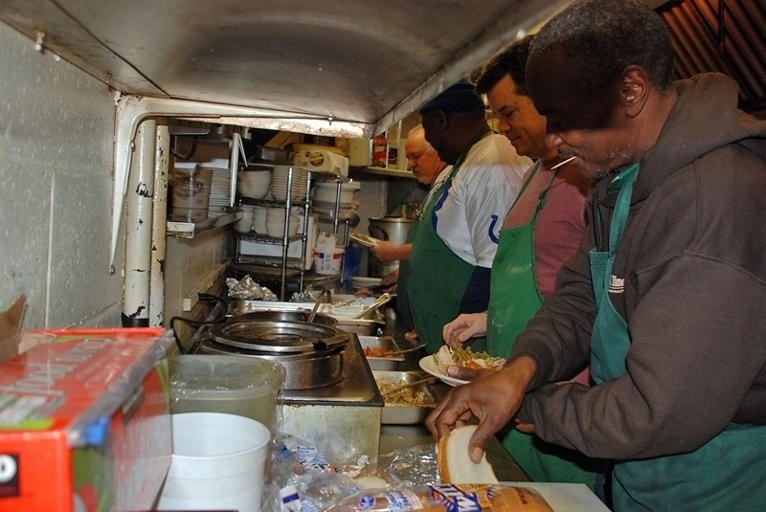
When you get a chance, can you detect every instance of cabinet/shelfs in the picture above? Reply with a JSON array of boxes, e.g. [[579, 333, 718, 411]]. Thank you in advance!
[[233, 166, 312, 278]]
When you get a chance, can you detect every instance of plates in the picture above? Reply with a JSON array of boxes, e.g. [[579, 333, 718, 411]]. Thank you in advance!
[[351, 276, 385, 290], [418, 353, 471, 388], [350, 232, 377, 248], [207, 166, 233, 209]]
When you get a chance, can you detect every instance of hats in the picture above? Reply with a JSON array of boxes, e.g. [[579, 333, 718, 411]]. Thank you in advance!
[[419, 78, 486, 113]]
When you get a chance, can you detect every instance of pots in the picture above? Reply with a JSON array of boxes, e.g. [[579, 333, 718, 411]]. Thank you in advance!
[[227, 308, 338, 327], [369, 213, 412, 279]]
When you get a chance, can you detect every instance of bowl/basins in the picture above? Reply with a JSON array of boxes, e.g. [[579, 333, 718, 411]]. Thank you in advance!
[[233, 165, 307, 237]]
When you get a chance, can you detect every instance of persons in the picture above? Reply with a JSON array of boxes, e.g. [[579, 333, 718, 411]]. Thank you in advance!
[[439, 33, 610, 504], [354, 122, 454, 328], [406, 78, 535, 354], [424, 0, 766, 512]]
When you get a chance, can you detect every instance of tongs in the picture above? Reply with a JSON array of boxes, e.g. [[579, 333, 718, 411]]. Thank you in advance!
[[306, 287, 333, 325]]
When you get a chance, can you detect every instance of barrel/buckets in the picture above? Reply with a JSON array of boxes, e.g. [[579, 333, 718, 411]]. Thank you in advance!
[[172, 166, 214, 223], [313, 247, 345, 276]]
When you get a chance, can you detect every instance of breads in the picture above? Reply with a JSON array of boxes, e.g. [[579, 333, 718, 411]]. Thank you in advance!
[[436, 425, 499, 485], [366, 484, 554, 512]]
